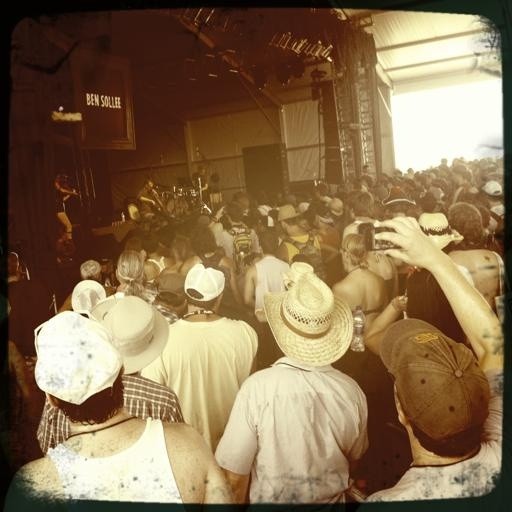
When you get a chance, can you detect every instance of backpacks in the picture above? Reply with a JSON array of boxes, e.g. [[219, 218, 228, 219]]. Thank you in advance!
[[228, 229, 255, 275], [283, 233, 332, 287]]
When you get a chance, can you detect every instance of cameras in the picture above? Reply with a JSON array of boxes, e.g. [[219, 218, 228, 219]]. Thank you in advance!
[[371, 227, 401, 251]]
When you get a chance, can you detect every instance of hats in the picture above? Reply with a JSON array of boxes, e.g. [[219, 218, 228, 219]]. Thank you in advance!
[[33, 310, 122, 406], [327, 198, 344, 216], [70, 279, 106, 315], [481, 180, 503, 196], [280, 261, 314, 289], [416, 213, 464, 251], [184, 263, 225, 303], [379, 319, 490, 437], [448, 251, 505, 311], [262, 272, 353, 366], [90, 296, 170, 375], [278, 204, 300, 222]]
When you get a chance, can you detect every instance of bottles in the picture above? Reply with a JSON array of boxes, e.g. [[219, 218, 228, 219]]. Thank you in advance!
[[349, 306, 366, 353]]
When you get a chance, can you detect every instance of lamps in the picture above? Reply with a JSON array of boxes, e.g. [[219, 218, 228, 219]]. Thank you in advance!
[[181, 7, 233, 68]]
